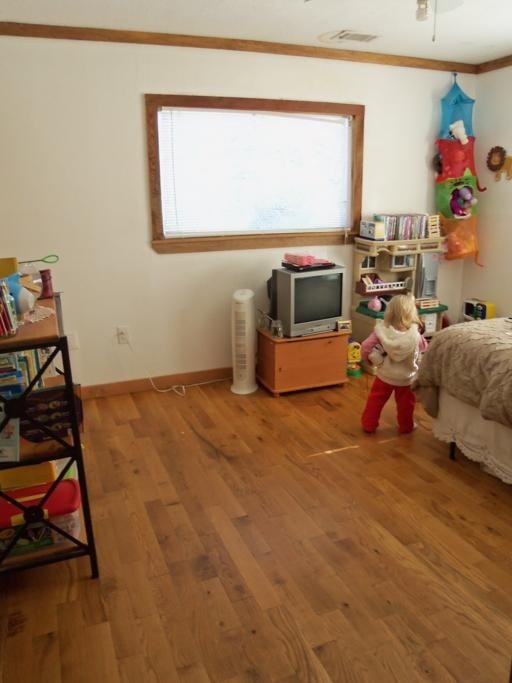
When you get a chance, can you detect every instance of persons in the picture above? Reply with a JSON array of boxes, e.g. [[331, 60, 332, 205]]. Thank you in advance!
[[360, 294, 429, 433]]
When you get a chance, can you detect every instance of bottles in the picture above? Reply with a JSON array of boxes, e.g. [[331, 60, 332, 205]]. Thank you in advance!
[[271, 320, 283, 339]]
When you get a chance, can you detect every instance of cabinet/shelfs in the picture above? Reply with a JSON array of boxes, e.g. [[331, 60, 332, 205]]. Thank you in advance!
[[352, 236, 449, 377], [0, 293, 100, 582], [256, 327, 353, 399]]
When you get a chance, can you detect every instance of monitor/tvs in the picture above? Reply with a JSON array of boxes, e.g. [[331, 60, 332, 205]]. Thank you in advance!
[[267, 265, 351, 338]]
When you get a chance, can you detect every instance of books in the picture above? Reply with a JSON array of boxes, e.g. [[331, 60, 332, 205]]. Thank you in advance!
[[374, 214, 427, 241], [0, 281, 55, 463]]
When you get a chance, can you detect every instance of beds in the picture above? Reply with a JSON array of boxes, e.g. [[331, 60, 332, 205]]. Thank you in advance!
[[415, 315, 510, 491]]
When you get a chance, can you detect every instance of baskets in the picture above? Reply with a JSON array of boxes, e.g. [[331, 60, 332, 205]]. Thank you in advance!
[[417, 297, 439, 308], [426, 214, 441, 238]]
